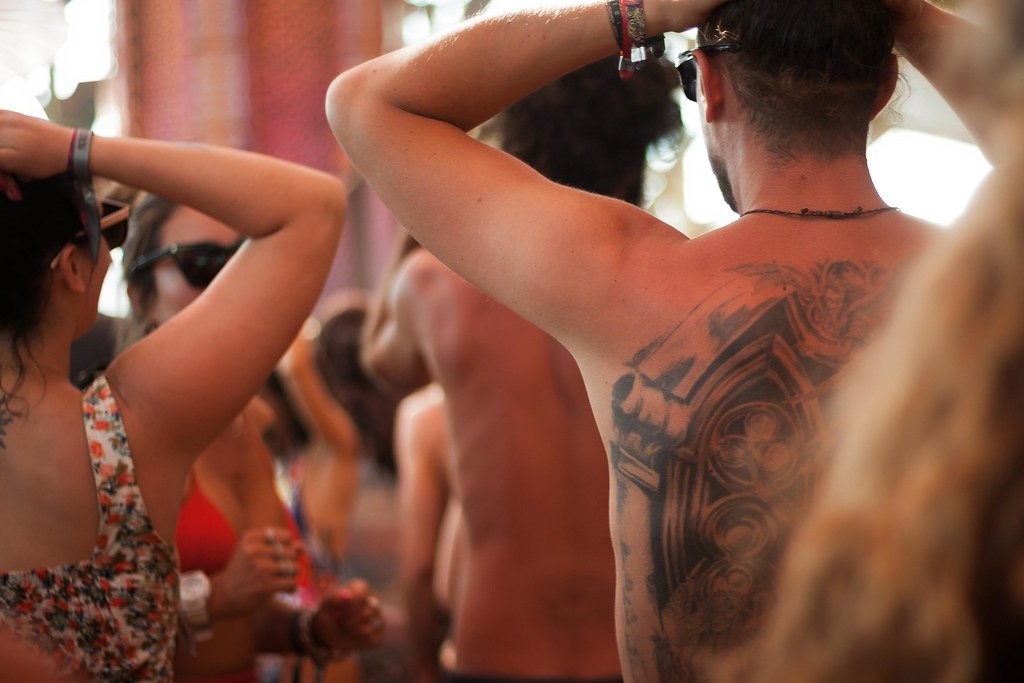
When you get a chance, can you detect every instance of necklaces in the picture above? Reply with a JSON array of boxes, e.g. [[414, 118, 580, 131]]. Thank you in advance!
[[742, 208, 904, 219]]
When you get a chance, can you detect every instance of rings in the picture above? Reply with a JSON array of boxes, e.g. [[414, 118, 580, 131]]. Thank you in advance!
[[265, 525, 278, 543], [278, 560, 294, 576], [273, 542, 285, 559]]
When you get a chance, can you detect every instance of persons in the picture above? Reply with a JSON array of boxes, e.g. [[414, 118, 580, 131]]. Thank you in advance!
[[0, 45, 684, 683], [325, 0, 1024, 683]]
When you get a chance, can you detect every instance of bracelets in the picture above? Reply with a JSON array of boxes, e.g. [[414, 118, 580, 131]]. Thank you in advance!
[[299, 608, 348, 665], [69, 128, 101, 274], [606, 0, 653, 80]]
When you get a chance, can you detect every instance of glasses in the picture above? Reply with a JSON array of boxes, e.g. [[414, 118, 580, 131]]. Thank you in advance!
[[125, 240, 245, 289], [675, 42, 740, 101], [50, 197, 129, 267]]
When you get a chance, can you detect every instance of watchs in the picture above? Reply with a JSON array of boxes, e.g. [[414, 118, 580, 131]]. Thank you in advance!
[[178, 569, 212, 645]]
